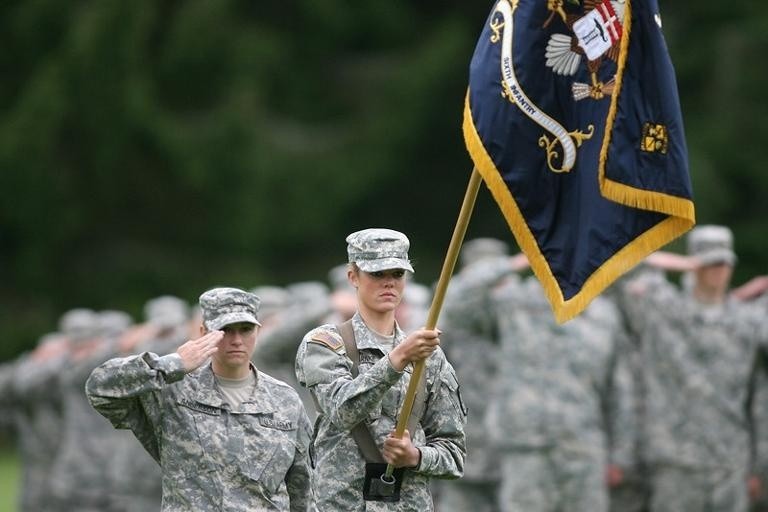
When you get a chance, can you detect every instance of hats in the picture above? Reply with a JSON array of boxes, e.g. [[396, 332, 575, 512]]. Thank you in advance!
[[285, 280, 332, 308], [329, 262, 365, 294], [94, 308, 134, 338], [457, 236, 511, 269], [198, 286, 263, 333], [345, 227, 416, 275], [58, 305, 102, 341], [401, 281, 433, 308], [686, 222, 738, 268], [251, 284, 294, 315], [140, 295, 190, 326]]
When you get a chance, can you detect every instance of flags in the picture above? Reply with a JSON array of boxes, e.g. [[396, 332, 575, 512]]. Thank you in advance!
[[461, 1, 695, 327]]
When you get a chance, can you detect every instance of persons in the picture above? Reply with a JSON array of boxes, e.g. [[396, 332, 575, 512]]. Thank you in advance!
[[250, 263, 358, 424], [295, 228, 469, 510], [397, 223, 768, 511], [85, 288, 314, 511], [1, 296, 201, 509]]
